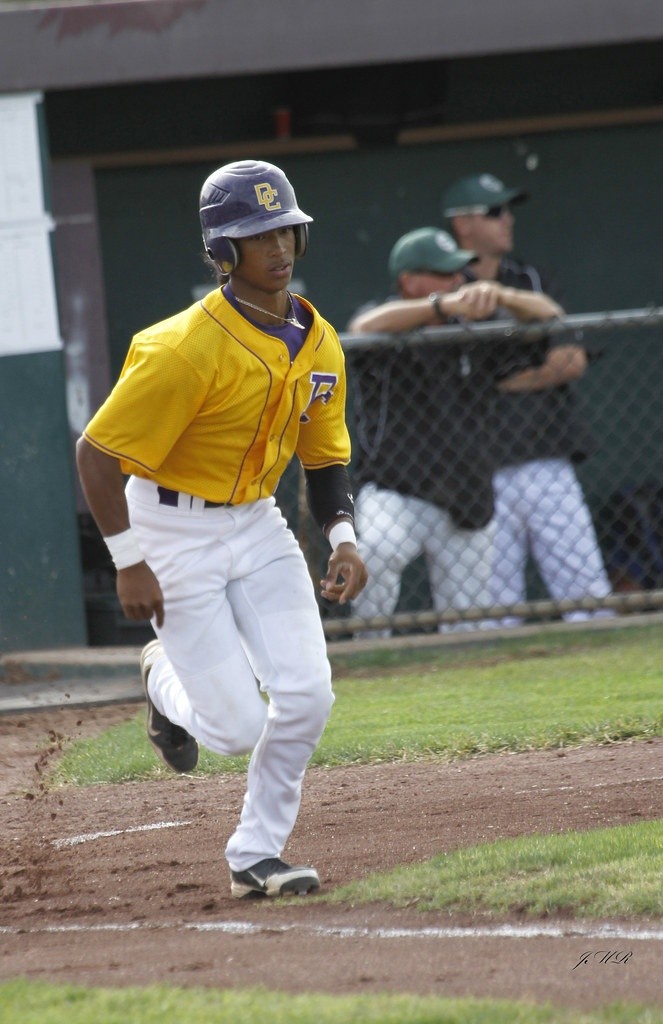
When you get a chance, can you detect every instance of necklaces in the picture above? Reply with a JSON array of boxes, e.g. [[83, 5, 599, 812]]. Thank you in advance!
[[235, 292, 305, 330]]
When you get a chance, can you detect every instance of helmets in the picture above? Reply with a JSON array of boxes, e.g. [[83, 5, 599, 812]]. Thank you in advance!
[[199, 159, 313, 275]]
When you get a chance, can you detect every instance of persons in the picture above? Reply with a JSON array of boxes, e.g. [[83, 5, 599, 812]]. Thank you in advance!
[[442, 173, 615, 627], [76, 159, 367, 900], [349, 225, 564, 640]]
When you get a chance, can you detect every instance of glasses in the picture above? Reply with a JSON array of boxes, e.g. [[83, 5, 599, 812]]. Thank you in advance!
[[479, 207, 503, 217]]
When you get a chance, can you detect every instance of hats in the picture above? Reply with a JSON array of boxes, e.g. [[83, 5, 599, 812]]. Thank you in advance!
[[445, 174, 527, 215], [389, 228, 480, 280]]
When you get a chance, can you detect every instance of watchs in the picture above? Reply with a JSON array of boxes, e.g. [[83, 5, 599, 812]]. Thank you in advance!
[[429, 292, 443, 316]]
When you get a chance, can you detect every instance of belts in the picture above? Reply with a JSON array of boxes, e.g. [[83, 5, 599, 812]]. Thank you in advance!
[[157, 484, 232, 509]]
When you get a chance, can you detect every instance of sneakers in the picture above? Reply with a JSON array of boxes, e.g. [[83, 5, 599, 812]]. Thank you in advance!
[[229, 858, 321, 900], [139, 639, 199, 774]]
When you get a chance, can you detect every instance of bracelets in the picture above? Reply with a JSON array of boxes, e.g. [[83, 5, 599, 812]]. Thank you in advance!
[[103, 527, 146, 571], [329, 522, 357, 552]]
